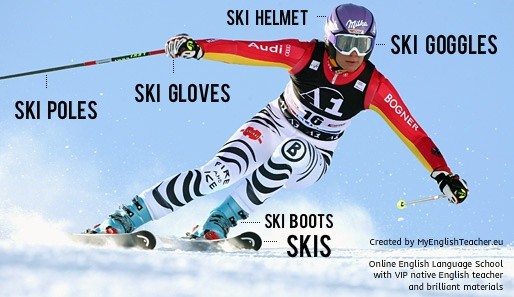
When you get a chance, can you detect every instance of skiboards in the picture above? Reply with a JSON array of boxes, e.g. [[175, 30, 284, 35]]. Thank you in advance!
[[69, 230, 262, 252]]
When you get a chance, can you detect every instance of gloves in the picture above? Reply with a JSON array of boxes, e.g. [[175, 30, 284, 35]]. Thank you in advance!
[[164, 33, 203, 60], [430, 167, 469, 204]]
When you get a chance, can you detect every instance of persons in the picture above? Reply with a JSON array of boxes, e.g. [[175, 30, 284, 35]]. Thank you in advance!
[[84, 3, 470, 240]]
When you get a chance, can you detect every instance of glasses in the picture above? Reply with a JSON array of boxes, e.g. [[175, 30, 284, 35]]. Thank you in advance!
[[326, 31, 374, 56]]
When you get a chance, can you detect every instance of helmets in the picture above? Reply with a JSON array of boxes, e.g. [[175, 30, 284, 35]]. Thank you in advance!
[[324, 4, 376, 60]]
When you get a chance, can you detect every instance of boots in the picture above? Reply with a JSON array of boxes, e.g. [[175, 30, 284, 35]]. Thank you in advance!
[[92, 194, 152, 233], [193, 193, 249, 238]]
